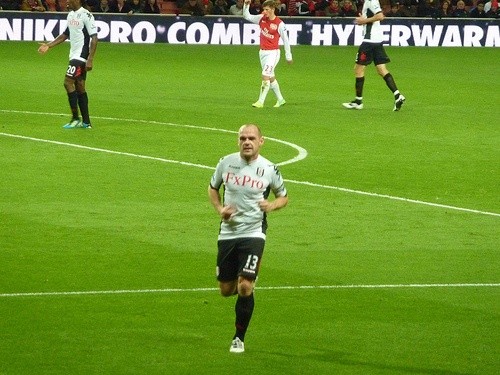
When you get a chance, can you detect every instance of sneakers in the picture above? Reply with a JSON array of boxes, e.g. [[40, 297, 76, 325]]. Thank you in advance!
[[75, 121, 92, 129], [393, 94, 405, 111], [62, 119, 83, 129], [342, 101, 364, 110], [229, 336, 245, 353], [273, 98, 286, 108], [252, 101, 263, 108]]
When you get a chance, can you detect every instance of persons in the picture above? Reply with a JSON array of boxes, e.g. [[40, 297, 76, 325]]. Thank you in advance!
[[0, 0, 244, 17], [342, 0, 405, 111], [243, 0, 292, 108], [275, 0, 364, 17], [209, 123, 288, 353], [379, 0, 500, 18], [37, 0, 98, 128]]
